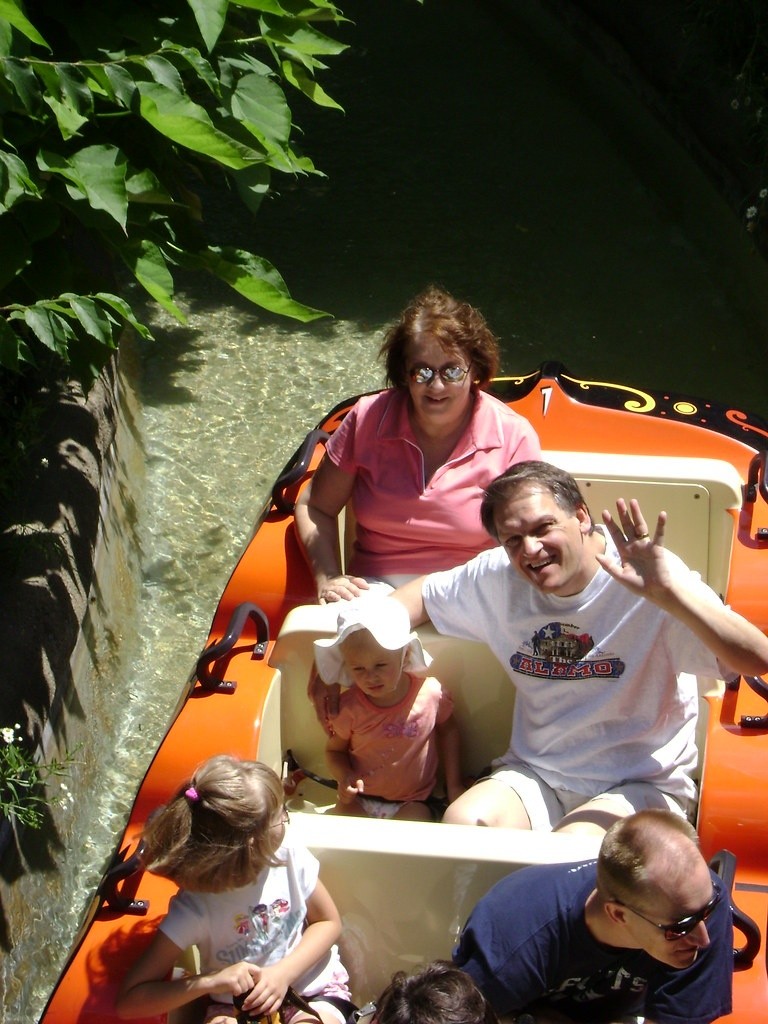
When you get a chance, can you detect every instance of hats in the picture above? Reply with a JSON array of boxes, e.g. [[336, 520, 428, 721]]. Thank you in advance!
[[313, 597, 434, 687]]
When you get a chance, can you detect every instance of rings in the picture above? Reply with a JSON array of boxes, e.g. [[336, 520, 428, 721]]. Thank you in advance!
[[636, 533, 650, 538]]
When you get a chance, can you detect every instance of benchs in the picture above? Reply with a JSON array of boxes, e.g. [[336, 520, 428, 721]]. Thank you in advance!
[[281, 449, 741, 1020]]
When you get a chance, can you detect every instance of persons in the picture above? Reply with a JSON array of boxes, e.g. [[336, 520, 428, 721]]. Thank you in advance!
[[113, 754, 353, 1024], [365, 958, 490, 1024], [308, 460, 768, 838], [313, 595, 469, 821], [452, 807, 734, 1024], [293, 285, 544, 607]]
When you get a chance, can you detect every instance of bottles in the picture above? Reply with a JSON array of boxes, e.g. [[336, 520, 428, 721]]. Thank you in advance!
[[233, 974, 284, 1024]]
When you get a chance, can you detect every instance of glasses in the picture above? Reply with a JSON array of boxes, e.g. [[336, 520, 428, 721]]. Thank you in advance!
[[267, 803, 290, 828], [610, 880, 722, 941], [404, 358, 474, 384]]
